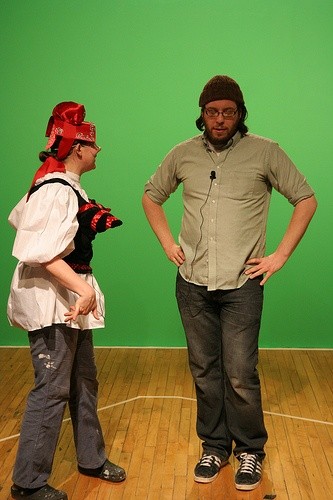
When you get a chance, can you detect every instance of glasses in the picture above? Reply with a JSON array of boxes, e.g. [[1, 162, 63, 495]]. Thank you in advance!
[[72, 143, 103, 155], [201, 105, 239, 118]]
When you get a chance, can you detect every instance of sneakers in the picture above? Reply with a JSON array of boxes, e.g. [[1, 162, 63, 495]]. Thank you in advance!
[[193, 450, 228, 483], [233, 453, 266, 490]]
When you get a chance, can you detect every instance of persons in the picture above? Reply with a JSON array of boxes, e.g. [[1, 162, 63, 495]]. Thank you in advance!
[[142, 75, 318, 490], [7, 102, 126, 500]]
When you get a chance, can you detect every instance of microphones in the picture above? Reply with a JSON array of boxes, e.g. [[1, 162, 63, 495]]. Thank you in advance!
[[210, 171, 216, 179], [91, 199, 95, 204]]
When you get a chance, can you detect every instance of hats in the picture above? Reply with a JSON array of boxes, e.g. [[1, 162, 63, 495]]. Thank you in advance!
[[197, 75, 245, 107], [45, 101, 96, 157]]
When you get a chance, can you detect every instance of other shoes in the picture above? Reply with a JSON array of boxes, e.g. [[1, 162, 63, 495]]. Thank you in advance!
[[77, 460, 126, 485], [11, 484, 66, 500]]
